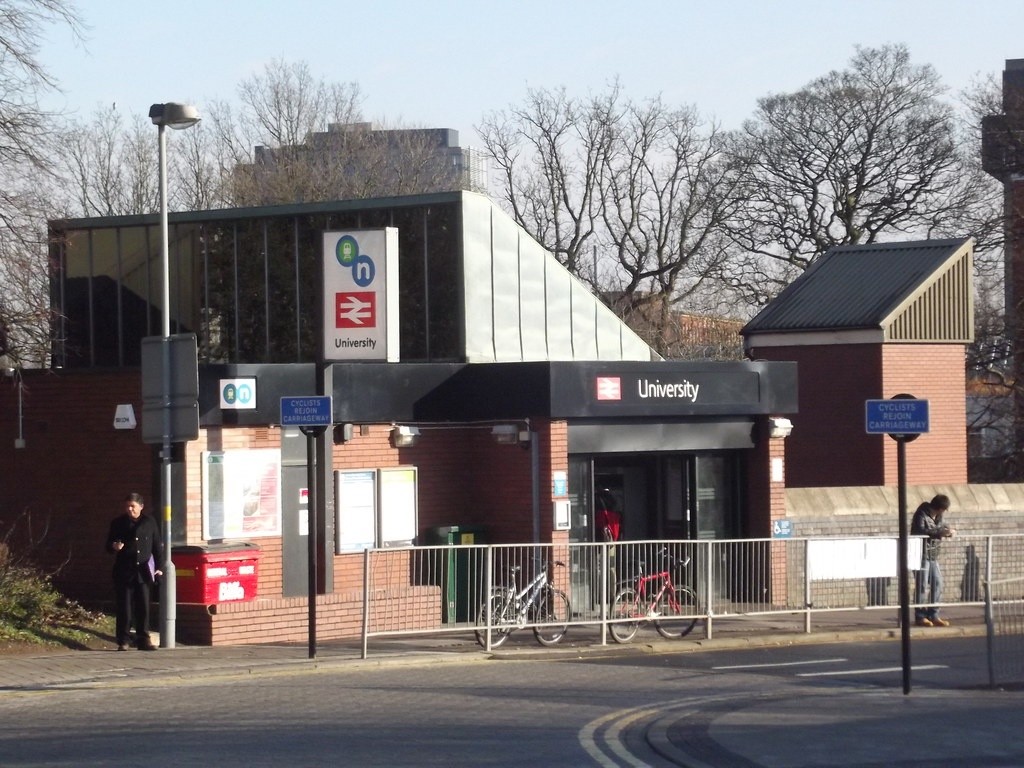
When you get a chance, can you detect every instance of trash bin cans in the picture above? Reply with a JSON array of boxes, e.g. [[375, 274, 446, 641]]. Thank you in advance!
[[424, 525, 494, 624]]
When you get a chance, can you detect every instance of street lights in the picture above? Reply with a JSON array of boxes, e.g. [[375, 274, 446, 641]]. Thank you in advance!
[[143, 99, 204, 649]]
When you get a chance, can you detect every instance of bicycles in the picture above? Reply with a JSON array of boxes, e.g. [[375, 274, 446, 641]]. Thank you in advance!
[[475, 558, 574, 650], [608, 546, 703, 643]]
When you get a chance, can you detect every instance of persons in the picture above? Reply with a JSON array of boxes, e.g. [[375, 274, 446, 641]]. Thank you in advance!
[[106, 492, 164, 652], [910, 494, 953, 627]]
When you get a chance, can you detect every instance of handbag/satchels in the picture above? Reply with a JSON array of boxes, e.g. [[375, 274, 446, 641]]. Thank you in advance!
[[596, 525, 613, 553]]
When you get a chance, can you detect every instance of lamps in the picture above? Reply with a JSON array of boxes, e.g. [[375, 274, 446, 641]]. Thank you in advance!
[[392, 426, 425, 447], [770, 418, 795, 437], [493, 425, 522, 446]]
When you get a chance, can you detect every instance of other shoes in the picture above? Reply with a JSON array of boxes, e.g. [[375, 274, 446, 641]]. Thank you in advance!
[[118, 642, 130, 651], [930, 617, 950, 627], [138, 643, 157, 651], [915, 617, 933, 627]]
